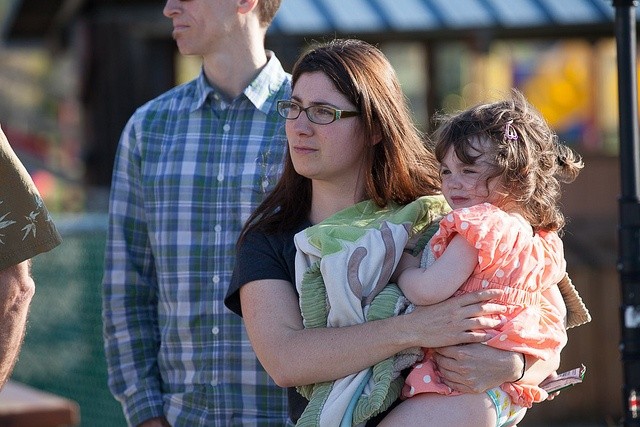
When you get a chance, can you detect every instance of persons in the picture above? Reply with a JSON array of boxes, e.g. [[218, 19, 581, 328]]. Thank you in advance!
[[101, 0, 311, 427], [221, 39, 561, 427], [375, 90, 585, 427], [0, 125, 63, 386]]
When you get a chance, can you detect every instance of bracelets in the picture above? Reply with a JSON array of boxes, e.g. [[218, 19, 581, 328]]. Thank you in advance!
[[513, 353, 526, 382]]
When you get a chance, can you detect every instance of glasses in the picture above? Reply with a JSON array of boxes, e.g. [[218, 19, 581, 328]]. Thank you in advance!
[[276, 100, 360, 126]]
